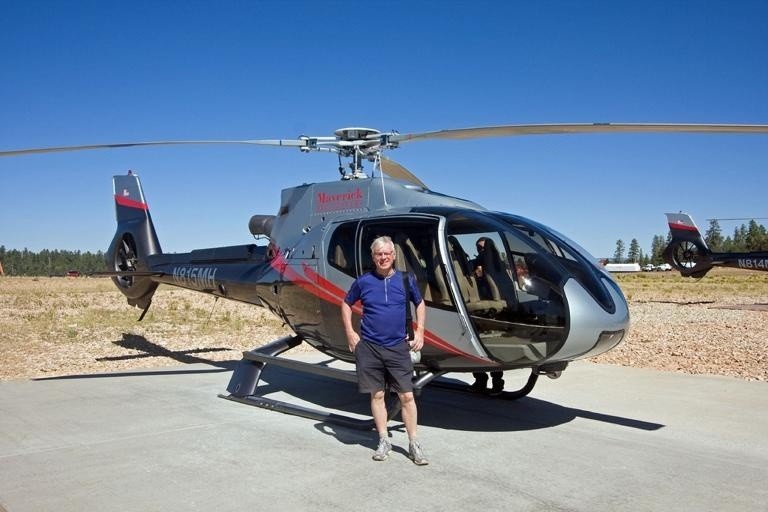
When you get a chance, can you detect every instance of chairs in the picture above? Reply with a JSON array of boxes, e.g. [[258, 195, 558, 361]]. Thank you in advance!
[[432, 236, 507, 316], [476, 235, 540, 305], [334, 235, 368, 270], [391, 233, 434, 303]]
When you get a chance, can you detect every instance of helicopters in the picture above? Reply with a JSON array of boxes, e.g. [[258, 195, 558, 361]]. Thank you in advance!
[[662, 212, 768, 278], [0, 124, 768, 431]]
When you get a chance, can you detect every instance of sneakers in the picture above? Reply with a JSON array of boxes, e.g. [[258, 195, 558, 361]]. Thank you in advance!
[[489, 388, 502, 395], [468, 384, 486, 391], [409, 443, 428, 464], [373, 437, 391, 460]]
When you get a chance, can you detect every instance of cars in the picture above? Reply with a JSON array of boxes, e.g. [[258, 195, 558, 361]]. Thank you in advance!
[[65, 270, 82, 277]]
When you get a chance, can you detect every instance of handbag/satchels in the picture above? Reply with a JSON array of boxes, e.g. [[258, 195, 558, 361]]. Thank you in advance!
[[406, 331, 422, 364]]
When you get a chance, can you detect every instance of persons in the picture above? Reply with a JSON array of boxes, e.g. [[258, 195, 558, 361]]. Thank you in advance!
[[340, 234, 430, 465], [470, 237, 505, 398]]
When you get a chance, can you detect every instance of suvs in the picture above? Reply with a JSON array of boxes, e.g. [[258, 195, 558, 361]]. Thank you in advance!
[[656, 263, 672, 272], [641, 263, 656, 272]]
